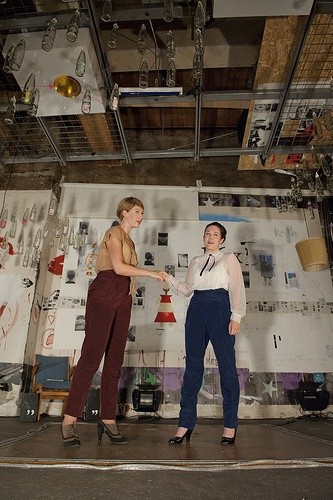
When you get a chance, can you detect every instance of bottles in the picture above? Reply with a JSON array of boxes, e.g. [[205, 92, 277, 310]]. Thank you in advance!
[[195, 2, 205, 28], [108, 24, 118, 48], [274, 108, 333, 214], [4, 99, 17, 124], [162, 0, 173, 22], [102, 0, 112, 22], [136, 25, 146, 55], [10, 39, 25, 71], [81, 88, 90, 113], [139, 60, 148, 89], [28, 89, 40, 115], [194, 29, 204, 56], [193, 55, 201, 79], [167, 59, 176, 86], [0, 193, 87, 268], [3, 43, 15, 72], [109, 84, 119, 110], [75, 51, 86, 77], [41, 19, 57, 52], [167, 31, 175, 58], [66, 10, 81, 42], [21, 74, 35, 104]]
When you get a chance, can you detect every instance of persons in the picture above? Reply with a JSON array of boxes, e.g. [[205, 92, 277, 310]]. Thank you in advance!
[[160, 222, 246, 447], [60, 197, 166, 447]]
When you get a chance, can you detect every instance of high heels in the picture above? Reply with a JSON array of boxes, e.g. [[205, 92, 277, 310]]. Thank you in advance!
[[60, 424, 80, 448], [97, 420, 129, 444], [168, 429, 193, 444], [221, 427, 237, 446]]
